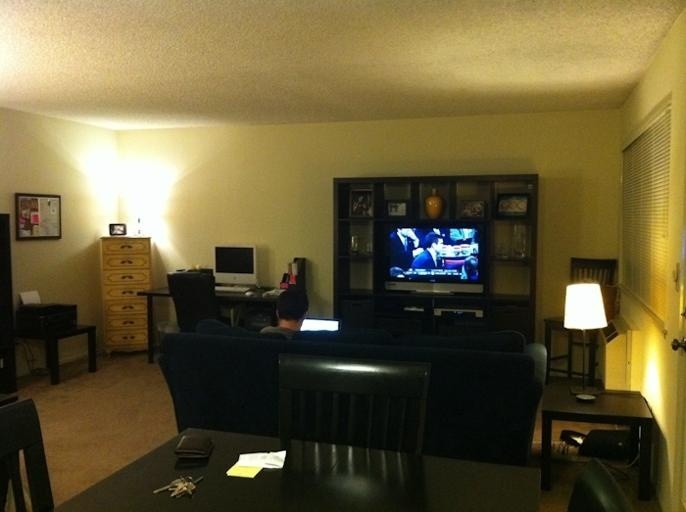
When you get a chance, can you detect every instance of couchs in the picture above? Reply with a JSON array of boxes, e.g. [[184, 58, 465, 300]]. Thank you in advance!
[[157, 318, 548, 465]]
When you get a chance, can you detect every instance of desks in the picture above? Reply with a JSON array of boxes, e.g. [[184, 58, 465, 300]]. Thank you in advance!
[[13, 324, 97, 385], [137, 284, 285, 364], [46, 428, 542, 512]]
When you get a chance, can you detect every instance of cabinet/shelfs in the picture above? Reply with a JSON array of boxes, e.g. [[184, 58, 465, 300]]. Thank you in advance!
[[332, 173, 539, 344], [99, 236, 153, 354]]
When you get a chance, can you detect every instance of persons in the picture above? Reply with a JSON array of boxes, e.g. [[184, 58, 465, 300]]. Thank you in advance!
[[259, 287, 310, 335], [463, 256, 479, 281], [421, 228, 455, 246], [449, 228, 477, 245], [389, 228, 419, 272], [410, 233, 446, 269]]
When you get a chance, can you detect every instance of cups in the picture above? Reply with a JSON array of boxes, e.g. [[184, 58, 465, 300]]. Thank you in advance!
[[495, 244, 510, 260]]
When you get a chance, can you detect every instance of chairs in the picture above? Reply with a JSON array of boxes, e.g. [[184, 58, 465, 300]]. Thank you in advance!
[[0, 397, 56, 512], [568, 456, 632, 511], [543, 256, 619, 387], [278, 352, 431, 454]]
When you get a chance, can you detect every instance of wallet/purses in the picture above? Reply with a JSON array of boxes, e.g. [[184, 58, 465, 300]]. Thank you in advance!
[[173, 434, 216, 461]]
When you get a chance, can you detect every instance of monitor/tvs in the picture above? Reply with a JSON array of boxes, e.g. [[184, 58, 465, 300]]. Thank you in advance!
[[381, 220, 484, 297], [214, 244, 256, 285]]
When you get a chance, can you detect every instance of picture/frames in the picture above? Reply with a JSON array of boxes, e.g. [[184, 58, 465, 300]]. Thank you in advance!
[[109, 224, 126, 236], [15, 193, 62, 240]]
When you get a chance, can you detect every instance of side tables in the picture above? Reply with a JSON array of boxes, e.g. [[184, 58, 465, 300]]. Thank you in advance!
[[540, 386, 654, 500]]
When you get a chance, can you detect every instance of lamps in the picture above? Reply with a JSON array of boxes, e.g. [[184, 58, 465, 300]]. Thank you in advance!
[[564, 281, 608, 402]]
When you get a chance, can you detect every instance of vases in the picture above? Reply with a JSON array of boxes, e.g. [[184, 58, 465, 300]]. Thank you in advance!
[[426, 187, 442, 218]]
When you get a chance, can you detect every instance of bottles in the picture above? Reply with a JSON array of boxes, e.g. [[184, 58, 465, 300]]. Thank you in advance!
[[350, 234, 360, 255], [512, 221, 527, 261], [425, 187, 445, 219]]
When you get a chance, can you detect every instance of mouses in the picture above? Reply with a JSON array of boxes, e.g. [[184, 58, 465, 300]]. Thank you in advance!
[[245, 290, 255, 297]]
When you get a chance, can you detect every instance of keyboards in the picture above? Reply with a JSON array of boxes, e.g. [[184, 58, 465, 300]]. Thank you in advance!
[[214, 285, 251, 293]]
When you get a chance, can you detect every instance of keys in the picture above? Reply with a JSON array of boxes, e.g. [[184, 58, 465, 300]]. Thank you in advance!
[[152, 475, 204, 499]]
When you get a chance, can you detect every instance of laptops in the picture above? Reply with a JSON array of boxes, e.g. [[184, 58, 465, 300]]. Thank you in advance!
[[299, 317, 341, 332]]
